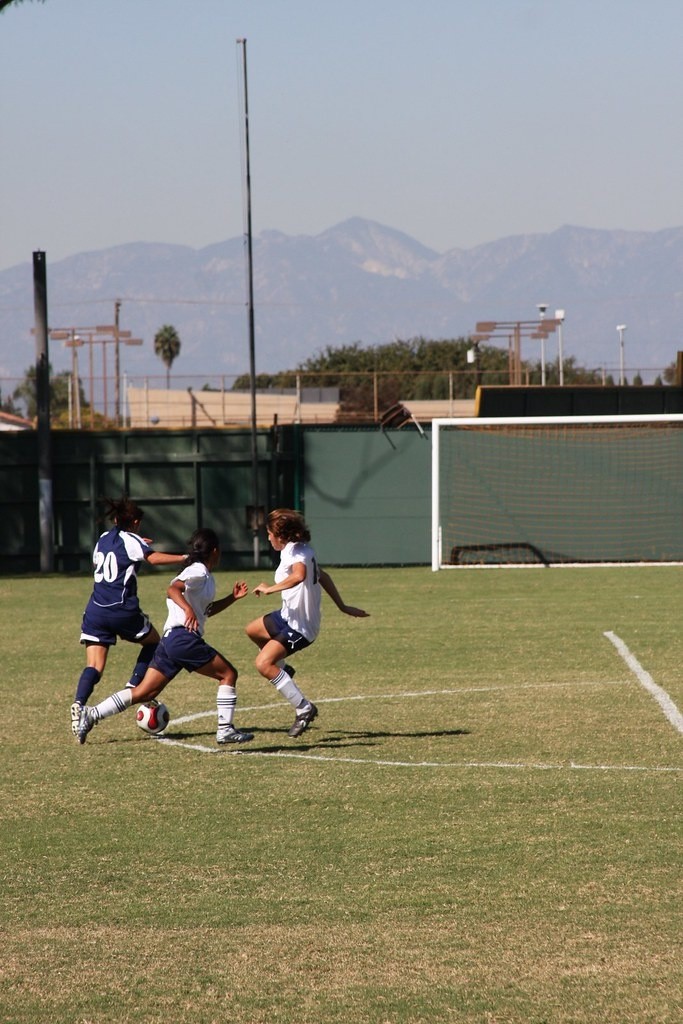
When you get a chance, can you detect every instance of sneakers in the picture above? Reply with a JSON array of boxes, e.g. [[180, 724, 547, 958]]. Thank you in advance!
[[216, 724, 254, 743], [71, 701, 83, 736], [284, 664, 296, 679], [123, 681, 159, 709], [288, 701, 318, 738], [77, 707, 98, 745]]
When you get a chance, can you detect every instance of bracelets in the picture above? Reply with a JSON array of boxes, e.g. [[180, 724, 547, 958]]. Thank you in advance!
[[183, 554, 189, 559]]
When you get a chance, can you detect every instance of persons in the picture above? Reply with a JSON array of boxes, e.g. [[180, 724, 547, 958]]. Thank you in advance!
[[246, 509, 371, 738], [71, 498, 189, 738], [76, 529, 256, 745]]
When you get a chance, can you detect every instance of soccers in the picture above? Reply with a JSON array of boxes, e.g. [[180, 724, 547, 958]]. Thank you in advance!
[[135, 700, 171, 735]]
